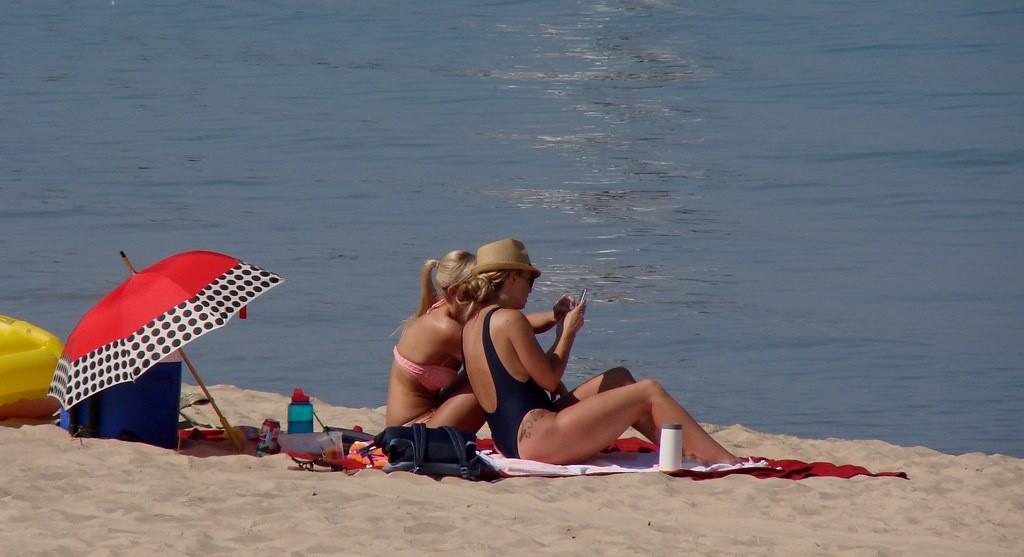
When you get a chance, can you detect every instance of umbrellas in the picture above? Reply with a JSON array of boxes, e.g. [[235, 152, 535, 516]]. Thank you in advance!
[[46, 249, 287, 450]]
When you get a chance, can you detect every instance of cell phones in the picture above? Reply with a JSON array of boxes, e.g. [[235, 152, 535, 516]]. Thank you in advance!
[[577, 289, 587, 305]]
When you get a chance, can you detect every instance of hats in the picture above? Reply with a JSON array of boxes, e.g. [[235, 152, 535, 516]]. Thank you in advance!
[[471, 239, 541, 278]]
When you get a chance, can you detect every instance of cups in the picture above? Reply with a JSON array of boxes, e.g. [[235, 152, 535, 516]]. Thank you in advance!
[[659, 424, 682, 471], [316, 432, 345, 472]]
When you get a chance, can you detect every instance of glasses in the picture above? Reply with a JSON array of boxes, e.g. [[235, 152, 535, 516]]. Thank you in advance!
[[514, 272, 534, 285]]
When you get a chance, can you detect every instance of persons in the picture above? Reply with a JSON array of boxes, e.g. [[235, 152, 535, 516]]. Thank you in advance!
[[384, 250, 577, 434], [449, 238, 739, 465]]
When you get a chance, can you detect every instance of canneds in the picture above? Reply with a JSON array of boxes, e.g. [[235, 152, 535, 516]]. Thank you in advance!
[[256, 418, 280, 453]]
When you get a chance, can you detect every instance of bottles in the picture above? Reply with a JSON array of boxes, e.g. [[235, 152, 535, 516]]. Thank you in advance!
[[286, 387, 313, 433]]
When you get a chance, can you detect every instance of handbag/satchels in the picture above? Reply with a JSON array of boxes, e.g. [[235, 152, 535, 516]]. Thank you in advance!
[[376, 422, 486, 479]]
